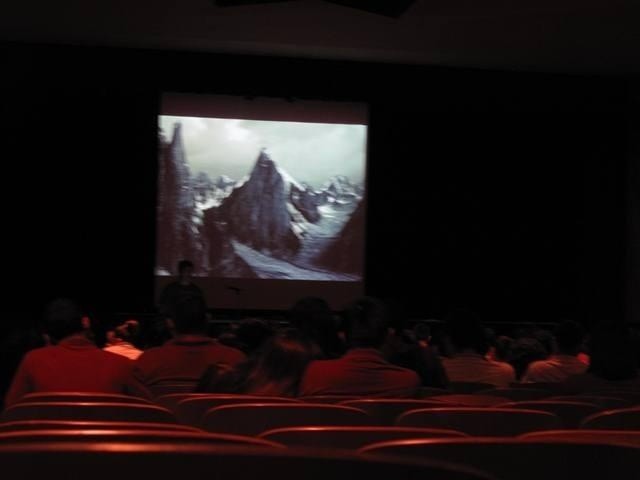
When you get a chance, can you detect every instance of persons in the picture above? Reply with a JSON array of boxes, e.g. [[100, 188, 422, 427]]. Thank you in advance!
[[158, 259, 213, 319]]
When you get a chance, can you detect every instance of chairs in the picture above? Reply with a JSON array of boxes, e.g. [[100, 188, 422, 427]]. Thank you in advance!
[[0, 376, 640, 480]]
